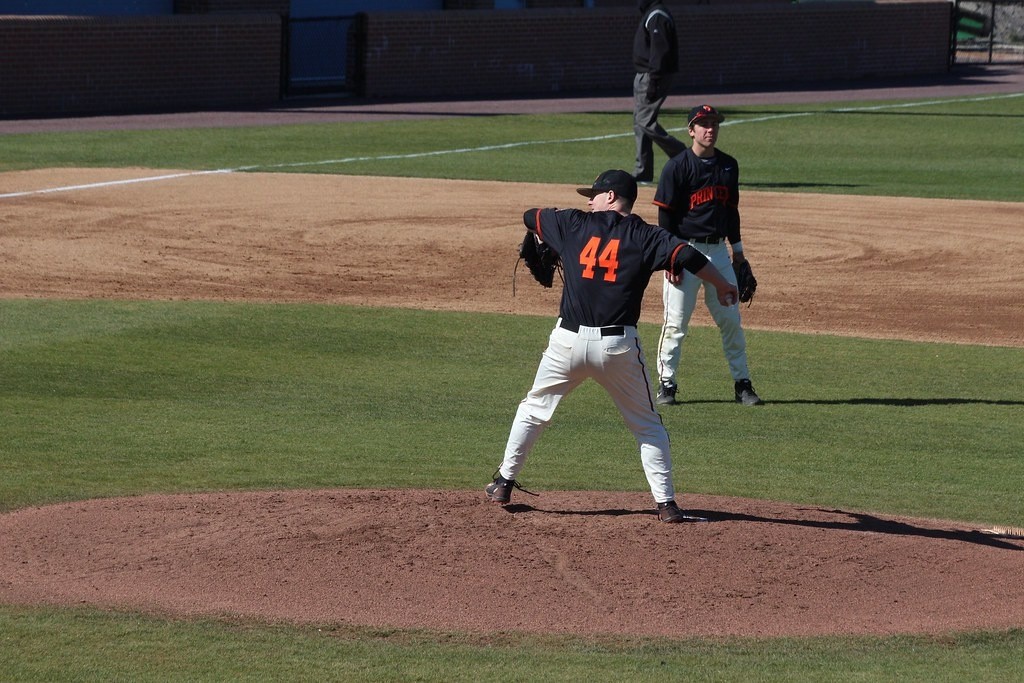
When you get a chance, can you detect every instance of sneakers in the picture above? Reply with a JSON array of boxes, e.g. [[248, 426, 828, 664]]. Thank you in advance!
[[734, 378, 761, 406], [485, 468, 540, 505], [656, 381, 680, 405], [656, 500, 683, 523]]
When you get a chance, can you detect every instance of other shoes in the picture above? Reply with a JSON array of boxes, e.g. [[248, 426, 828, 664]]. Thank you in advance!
[[630, 172, 653, 185]]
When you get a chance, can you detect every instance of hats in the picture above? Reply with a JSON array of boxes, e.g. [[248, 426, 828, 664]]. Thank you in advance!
[[688, 105, 725, 127], [576, 169, 637, 201]]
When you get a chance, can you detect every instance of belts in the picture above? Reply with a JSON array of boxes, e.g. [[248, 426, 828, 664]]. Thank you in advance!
[[560, 319, 637, 336], [677, 235, 720, 244]]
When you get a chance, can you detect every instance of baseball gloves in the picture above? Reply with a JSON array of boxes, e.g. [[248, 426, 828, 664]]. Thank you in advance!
[[519, 229, 560, 288], [732, 260, 757, 305]]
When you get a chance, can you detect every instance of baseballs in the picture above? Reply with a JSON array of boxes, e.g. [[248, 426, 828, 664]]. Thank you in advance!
[[723, 295, 735, 307]]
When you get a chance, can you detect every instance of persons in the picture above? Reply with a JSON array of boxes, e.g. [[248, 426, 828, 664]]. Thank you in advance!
[[652, 106, 766, 407], [485, 170, 738, 523], [631, 0, 687, 182]]
